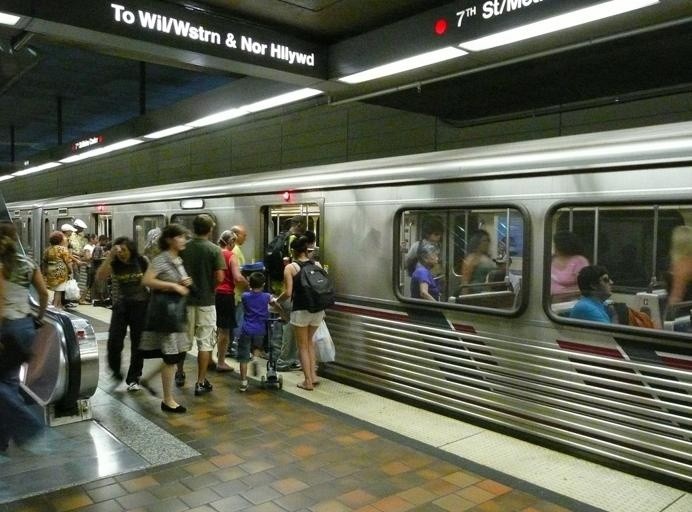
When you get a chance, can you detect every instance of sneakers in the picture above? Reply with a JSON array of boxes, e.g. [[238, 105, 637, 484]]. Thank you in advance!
[[239, 361, 259, 394], [277, 362, 303, 372], [64, 297, 114, 309], [161, 401, 188, 414], [175, 370, 187, 387], [194, 379, 214, 396], [128, 381, 142, 392], [207, 361, 235, 373]]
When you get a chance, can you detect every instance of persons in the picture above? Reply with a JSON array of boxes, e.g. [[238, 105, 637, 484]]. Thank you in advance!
[[459, 228, 495, 294], [567, 264, 622, 321], [0, 234, 49, 452], [666, 223, 691, 313], [411, 243, 442, 299], [489, 253, 519, 294], [550, 230, 589, 296], [402, 217, 442, 277]]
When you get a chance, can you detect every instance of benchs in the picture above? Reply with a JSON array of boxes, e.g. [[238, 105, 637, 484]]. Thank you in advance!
[[447, 276, 690, 335]]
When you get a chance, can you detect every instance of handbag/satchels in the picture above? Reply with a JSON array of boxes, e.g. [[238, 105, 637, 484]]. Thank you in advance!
[[64, 277, 80, 300], [143, 288, 189, 333]]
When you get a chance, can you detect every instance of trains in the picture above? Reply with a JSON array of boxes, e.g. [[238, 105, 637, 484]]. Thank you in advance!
[[5, 119, 691, 496]]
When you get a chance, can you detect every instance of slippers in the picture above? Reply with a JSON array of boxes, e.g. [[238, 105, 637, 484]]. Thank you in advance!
[[297, 379, 321, 392]]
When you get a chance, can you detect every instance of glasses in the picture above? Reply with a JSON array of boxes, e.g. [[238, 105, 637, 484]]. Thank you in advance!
[[430, 231, 444, 235]]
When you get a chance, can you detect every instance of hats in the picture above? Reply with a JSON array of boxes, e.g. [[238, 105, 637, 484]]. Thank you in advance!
[[144, 227, 163, 249], [494, 253, 512, 263], [61, 218, 88, 233], [193, 213, 217, 228]]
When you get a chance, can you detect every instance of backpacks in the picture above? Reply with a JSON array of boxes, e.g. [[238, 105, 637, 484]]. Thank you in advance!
[[266, 231, 294, 283], [292, 258, 336, 314]]
